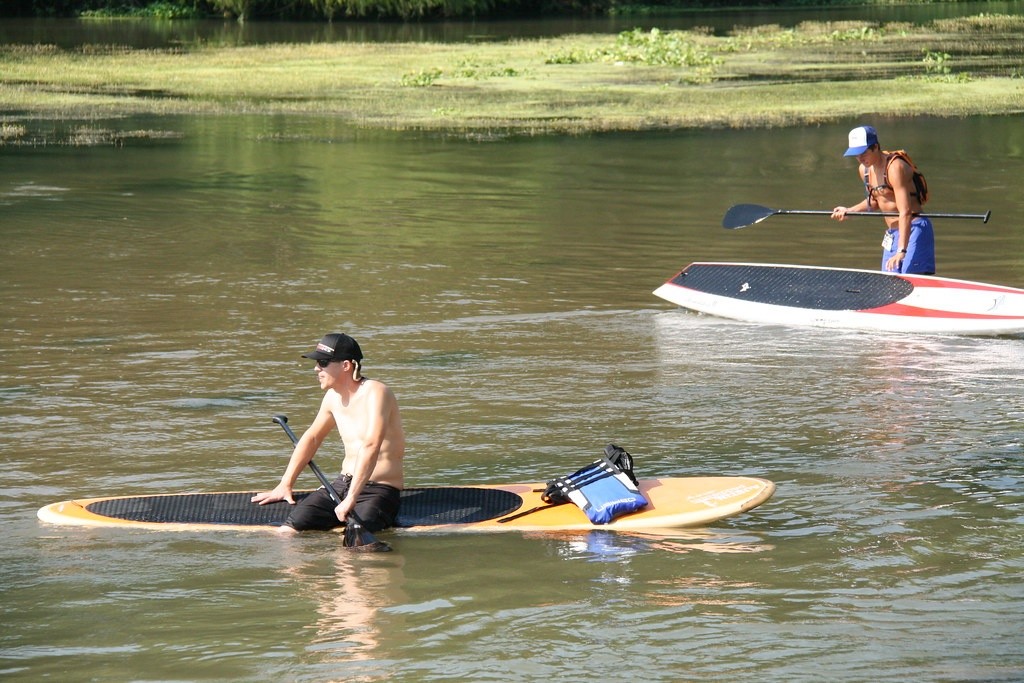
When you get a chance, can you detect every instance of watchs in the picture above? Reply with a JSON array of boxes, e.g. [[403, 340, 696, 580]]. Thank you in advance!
[[897, 249, 907, 253]]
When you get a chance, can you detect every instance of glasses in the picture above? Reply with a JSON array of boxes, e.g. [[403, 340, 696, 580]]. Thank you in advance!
[[311, 357, 335, 372]]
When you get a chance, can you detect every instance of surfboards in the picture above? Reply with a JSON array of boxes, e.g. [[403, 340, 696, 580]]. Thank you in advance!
[[651, 261, 1024, 335], [36, 474, 775, 538]]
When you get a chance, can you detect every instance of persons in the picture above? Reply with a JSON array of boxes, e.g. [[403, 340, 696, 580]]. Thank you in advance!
[[252, 333, 404, 535], [831, 125, 936, 275]]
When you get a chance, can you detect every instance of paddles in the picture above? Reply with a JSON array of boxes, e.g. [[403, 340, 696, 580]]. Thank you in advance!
[[722, 202, 988, 230], [272, 415, 391, 551]]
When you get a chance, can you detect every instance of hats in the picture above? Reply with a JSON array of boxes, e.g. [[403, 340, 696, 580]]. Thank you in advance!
[[842, 125, 880, 156], [298, 334, 366, 361]]
